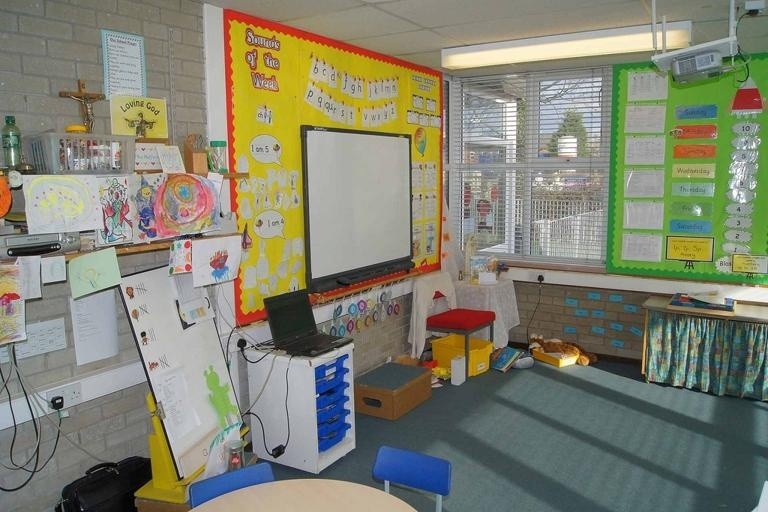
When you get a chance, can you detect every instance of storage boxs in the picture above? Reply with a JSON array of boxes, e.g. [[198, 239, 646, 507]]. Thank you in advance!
[[428, 332, 495, 377]]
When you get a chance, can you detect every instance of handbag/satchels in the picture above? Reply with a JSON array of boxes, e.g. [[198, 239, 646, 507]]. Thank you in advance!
[[55, 455, 152, 512]]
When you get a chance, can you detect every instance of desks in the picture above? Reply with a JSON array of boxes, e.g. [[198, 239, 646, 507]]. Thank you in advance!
[[187, 479, 418, 512], [641, 296, 768, 389]]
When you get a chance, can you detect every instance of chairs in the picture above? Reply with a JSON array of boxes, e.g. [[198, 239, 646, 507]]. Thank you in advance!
[[413, 272, 497, 383], [371, 443, 452, 511], [189, 461, 275, 509]]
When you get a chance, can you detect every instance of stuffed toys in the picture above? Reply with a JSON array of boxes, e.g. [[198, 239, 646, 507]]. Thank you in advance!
[[527, 335, 599, 366]]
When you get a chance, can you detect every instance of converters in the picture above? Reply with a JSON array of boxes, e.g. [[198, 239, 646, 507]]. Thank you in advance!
[[272, 445, 284, 457], [52, 396, 64, 409]]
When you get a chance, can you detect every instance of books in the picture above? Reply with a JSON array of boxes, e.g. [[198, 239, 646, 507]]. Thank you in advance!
[[669, 291, 735, 311], [489, 347, 523, 373]]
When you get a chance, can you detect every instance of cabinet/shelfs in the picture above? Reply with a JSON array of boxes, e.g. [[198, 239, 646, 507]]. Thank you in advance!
[[0, 170, 250, 269], [247, 339, 357, 475]]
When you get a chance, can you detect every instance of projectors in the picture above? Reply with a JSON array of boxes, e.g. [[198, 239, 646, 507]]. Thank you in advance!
[[672, 50, 721, 89]]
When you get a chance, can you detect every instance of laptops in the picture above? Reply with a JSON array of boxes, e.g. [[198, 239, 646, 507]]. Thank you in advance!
[[263, 288, 353, 357]]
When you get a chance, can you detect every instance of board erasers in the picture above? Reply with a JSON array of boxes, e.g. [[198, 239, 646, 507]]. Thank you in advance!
[[336, 277, 352, 285]]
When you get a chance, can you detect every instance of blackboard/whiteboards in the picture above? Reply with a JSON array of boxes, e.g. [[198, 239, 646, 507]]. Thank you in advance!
[[301, 125, 415, 293]]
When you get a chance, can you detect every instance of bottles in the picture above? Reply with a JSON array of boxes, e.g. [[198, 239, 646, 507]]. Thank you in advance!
[[210, 141, 228, 176], [0, 115, 23, 174], [58, 138, 102, 172]]
